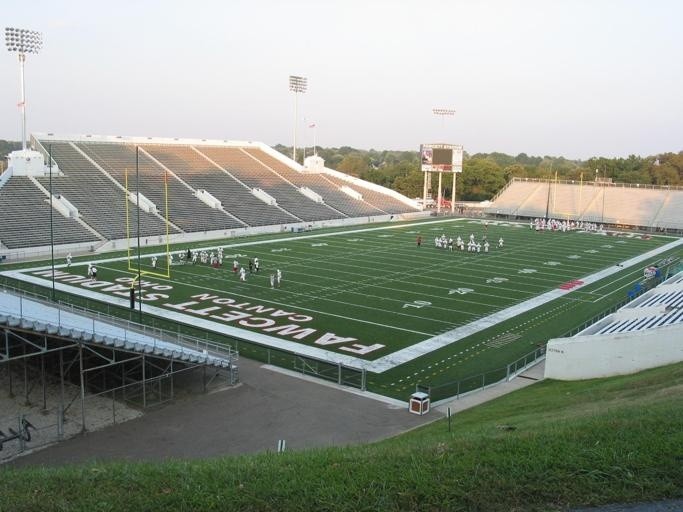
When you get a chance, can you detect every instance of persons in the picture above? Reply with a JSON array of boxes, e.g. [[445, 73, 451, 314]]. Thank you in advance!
[[270, 274, 274, 289], [167, 252, 173, 266], [85, 263, 98, 282], [642, 263, 661, 286], [415, 235, 422, 248], [151, 255, 157, 268], [529, 217, 603, 233], [484, 243, 488, 243], [178, 246, 259, 282], [275, 269, 281, 287], [66, 252, 72, 269], [434, 220, 504, 256]]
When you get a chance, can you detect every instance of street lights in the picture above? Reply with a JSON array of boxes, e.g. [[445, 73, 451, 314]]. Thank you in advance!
[[286, 73, 309, 162], [432, 107, 456, 141], [3, 26, 43, 152]]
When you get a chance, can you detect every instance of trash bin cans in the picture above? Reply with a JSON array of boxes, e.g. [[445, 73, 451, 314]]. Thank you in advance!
[[408, 391, 430, 416]]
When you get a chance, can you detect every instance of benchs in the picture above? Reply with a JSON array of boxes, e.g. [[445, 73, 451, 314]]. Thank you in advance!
[[486, 182, 683, 227], [0, 291, 232, 370], [594, 264, 682, 337]]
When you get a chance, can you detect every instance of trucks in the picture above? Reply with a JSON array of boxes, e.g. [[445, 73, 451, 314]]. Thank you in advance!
[[418, 198, 450, 208]]
